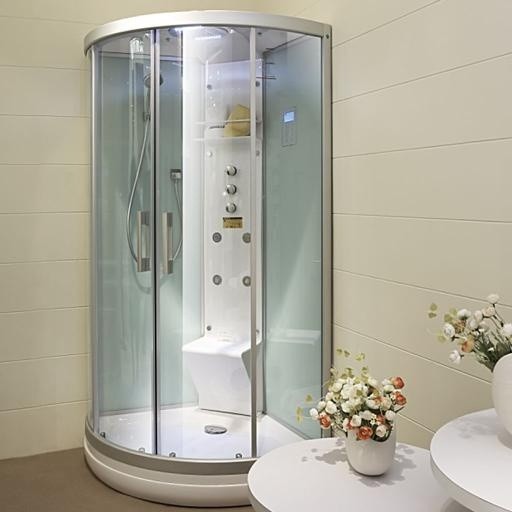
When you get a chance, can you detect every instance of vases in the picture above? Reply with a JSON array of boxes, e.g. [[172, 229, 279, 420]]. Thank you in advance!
[[492, 352, 512, 434]]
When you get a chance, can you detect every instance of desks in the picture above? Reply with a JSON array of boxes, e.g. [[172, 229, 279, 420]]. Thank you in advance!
[[247, 407, 512, 512]]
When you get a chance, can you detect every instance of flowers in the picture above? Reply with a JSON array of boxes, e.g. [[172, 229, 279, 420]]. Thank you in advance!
[[429, 294, 512, 372], [297, 349, 407, 441]]
